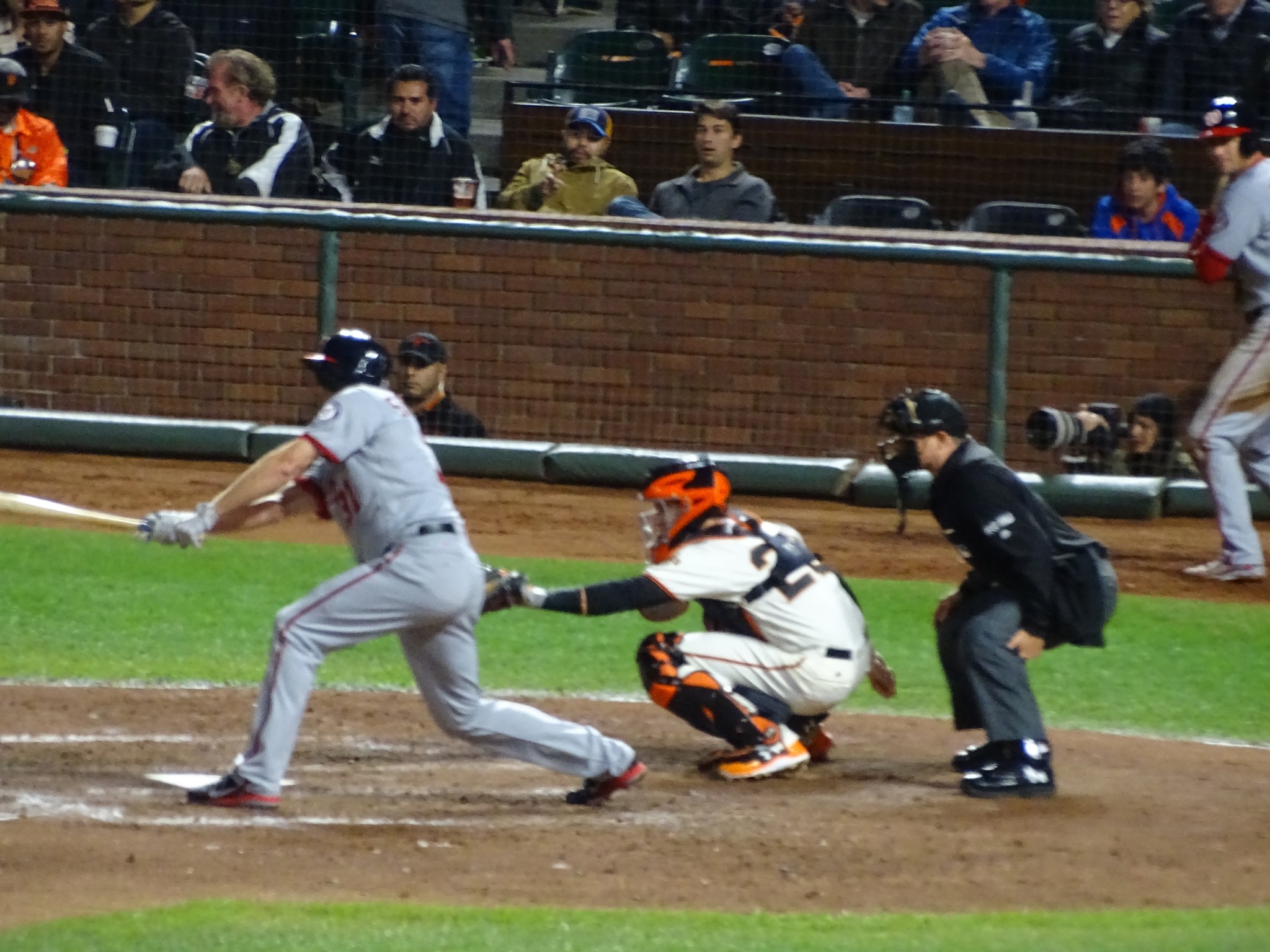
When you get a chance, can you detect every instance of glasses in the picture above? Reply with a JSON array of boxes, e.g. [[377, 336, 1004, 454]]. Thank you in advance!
[[398, 355, 430, 369]]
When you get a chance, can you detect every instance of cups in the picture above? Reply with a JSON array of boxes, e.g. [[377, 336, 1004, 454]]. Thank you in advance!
[[1139, 117, 1162, 147], [95, 125, 119, 148], [185, 75, 207, 100], [452, 177, 479, 209]]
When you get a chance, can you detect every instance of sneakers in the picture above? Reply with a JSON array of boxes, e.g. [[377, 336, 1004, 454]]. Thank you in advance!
[[567, 755, 648, 804], [715, 723, 812, 778], [789, 720, 834, 756], [1181, 547, 1268, 580], [186, 765, 283, 806]]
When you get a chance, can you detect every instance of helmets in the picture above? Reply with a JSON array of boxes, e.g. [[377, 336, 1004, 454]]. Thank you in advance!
[[643, 465, 730, 564], [1198, 95, 1254, 138], [883, 387, 967, 430], [300, 329, 391, 378]]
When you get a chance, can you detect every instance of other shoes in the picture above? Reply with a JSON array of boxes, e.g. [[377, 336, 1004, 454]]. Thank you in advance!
[[936, 88, 980, 127]]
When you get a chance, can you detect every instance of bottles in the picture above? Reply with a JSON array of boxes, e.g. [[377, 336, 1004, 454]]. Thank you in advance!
[[893, 90, 914, 123]]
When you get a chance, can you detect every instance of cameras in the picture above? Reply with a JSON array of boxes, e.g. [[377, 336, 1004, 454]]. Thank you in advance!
[[1025, 404, 1124, 452]]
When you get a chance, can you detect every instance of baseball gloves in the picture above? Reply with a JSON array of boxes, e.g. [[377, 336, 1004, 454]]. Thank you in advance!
[[459, 561, 526, 622]]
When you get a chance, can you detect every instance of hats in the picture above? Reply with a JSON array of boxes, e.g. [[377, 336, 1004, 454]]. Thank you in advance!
[[19, 0, 64, 13], [562, 104, 612, 140], [1129, 394, 1178, 430], [0, 57, 31, 101], [390, 332, 447, 359]]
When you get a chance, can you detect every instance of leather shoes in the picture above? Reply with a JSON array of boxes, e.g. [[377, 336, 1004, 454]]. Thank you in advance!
[[950, 733, 1053, 771], [959, 752, 1059, 798]]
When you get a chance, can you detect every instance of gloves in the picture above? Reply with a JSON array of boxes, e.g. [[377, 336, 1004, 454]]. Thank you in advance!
[[139, 509, 199, 541], [153, 502, 219, 549]]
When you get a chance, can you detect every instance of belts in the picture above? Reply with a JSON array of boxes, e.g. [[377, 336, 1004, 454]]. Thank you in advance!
[[823, 628, 869, 659], [381, 521, 456, 553], [1246, 306, 1265, 323]]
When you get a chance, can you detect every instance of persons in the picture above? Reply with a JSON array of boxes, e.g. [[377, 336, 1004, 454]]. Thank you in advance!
[[383, 331, 490, 479], [138, 326, 649, 806], [876, 389, 1121, 800], [1079, 388, 1203, 479], [482, 464, 896, 781], [1176, 96, 1270, 585], [0, 0, 1270, 243]]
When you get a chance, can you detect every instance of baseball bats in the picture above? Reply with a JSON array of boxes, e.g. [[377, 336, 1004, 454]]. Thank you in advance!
[[0, 488, 204, 546]]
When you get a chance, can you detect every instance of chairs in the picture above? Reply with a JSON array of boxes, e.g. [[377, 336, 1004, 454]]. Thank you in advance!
[[649, 31, 789, 113], [957, 200, 1090, 235], [812, 195, 947, 233], [97, 0, 358, 193], [539, 27, 680, 108]]
